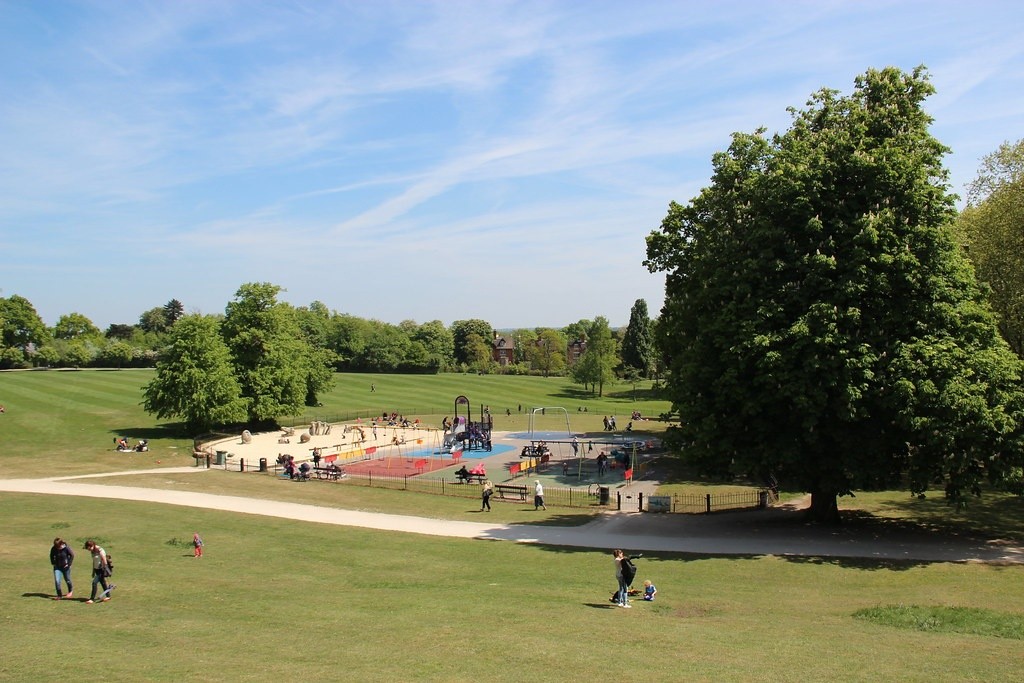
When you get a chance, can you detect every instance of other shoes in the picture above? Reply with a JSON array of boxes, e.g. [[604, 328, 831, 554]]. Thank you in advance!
[[650, 598, 654, 601], [618, 603, 624, 607], [86, 599, 93, 604], [480, 508, 484, 511], [53, 596, 63, 600], [486, 508, 491, 512], [199, 554, 202, 557], [102, 596, 111, 601], [65, 591, 72, 597], [534, 509, 538, 511], [194, 554, 198, 558], [543, 507, 546, 511], [623, 605, 632, 608]]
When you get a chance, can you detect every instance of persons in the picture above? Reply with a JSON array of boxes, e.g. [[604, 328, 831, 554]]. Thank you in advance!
[[459, 465, 492, 512], [610, 549, 657, 608], [355, 411, 420, 440], [84, 541, 111, 604], [119, 436, 147, 451], [370, 383, 376, 392], [506, 404, 642, 477], [193, 534, 204, 558], [278, 447, 340, 481], [534, 480, 547, 510], [50, 538, 74, 600], [443, 405, 493, 452]]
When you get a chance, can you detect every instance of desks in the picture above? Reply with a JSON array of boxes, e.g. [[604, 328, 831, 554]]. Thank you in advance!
[[521, 446, 537, 456]]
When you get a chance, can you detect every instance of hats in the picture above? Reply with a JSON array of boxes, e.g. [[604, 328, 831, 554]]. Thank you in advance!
[[194, 534, 198, 538], [534, 480, 540, 484]]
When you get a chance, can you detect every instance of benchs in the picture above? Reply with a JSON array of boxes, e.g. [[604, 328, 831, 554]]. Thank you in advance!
[[455, 472, 488, 485], [313, 466, 341, 480], [522, 449, 530, 452], [495, 485, 531, 501]]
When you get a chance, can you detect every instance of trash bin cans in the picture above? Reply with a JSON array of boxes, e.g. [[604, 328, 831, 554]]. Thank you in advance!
[[599, 487, 609, 505], [216, 450, 227, 465], [259, 458, 267, 472]]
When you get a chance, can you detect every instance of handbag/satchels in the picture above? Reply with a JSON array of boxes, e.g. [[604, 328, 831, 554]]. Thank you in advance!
[[481, 488, 493, 498], [98, 549, 113, 577]]
